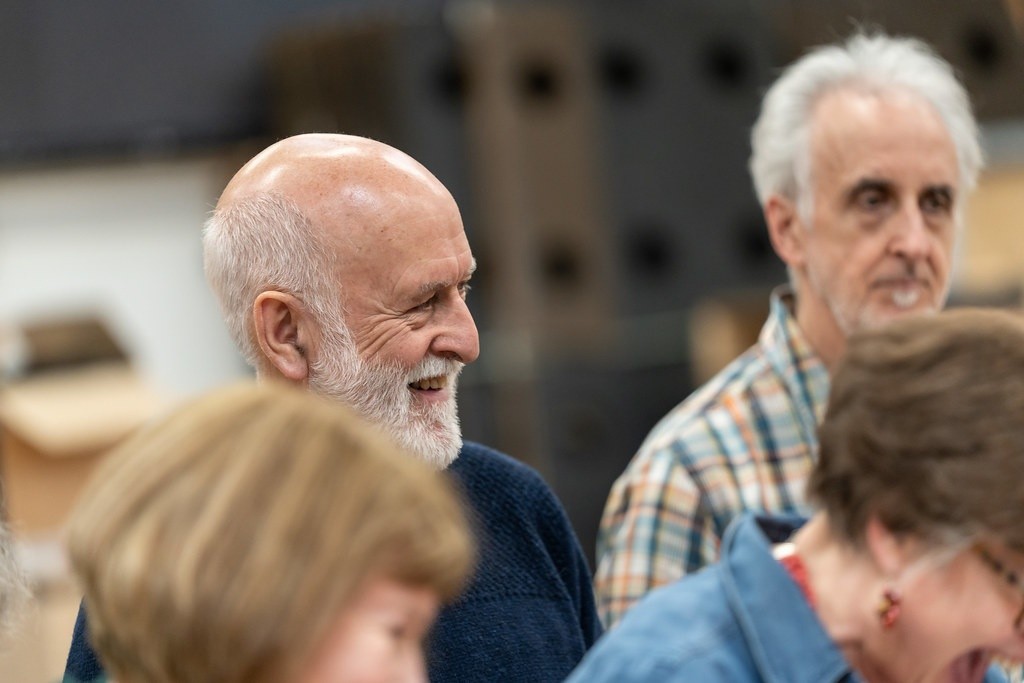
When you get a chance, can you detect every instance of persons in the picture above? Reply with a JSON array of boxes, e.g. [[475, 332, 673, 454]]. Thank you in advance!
[[561, 300, 1024, 683], [58, 131, 607, 683], [591, 30, 990, 634]]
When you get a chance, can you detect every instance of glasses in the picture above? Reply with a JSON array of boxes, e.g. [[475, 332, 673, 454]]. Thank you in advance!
[[965, 539, 1022, 636]]
